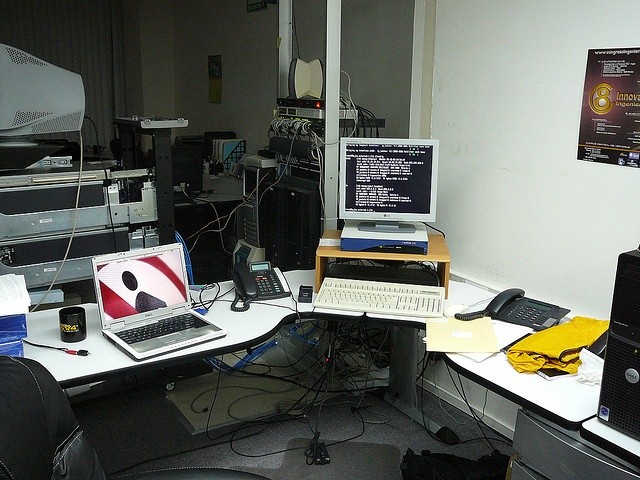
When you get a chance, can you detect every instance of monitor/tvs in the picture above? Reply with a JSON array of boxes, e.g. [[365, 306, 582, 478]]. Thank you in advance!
[[173, 147, 204, 202], [339, 137, 440, 234]]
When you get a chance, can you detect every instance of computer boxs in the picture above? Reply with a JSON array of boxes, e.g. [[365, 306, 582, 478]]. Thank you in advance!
[[238, 167, 278, 246], [596, 244, 640, 441]]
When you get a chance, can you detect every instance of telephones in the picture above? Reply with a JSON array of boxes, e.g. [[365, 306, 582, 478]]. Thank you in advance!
[[455, 288, 571, 331], [230, 259, 292, 312]]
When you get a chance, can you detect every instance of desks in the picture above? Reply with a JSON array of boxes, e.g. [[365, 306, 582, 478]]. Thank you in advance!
[[174, 170, 253, 206], [386, 262, 640, 480], [22, 267, 316, 388]]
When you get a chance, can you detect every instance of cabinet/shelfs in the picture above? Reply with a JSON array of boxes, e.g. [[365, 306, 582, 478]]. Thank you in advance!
[[274, 115, 386, 175]]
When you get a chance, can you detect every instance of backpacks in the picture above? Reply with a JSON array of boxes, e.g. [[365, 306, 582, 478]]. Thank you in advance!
[[400, 448, 510, 480]]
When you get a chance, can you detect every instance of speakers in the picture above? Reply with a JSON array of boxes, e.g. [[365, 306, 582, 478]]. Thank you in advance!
[[288, 58, 323, 99]]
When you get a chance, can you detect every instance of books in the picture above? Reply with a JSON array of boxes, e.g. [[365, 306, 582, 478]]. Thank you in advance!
[[213, 139, 244, 171], [502, 333, 578, 381]]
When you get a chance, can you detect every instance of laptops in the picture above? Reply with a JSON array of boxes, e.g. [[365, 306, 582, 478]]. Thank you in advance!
[[91, 243, 227, 362]]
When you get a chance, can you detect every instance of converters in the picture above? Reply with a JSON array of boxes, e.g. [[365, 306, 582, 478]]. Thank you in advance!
[[311, 442, 330, 464]]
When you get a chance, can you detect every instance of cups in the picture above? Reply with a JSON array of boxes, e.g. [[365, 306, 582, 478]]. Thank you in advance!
[[59, 307, 86, 343]]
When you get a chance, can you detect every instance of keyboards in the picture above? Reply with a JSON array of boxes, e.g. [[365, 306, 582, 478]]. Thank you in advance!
[[313, 277, 446, 318]]
